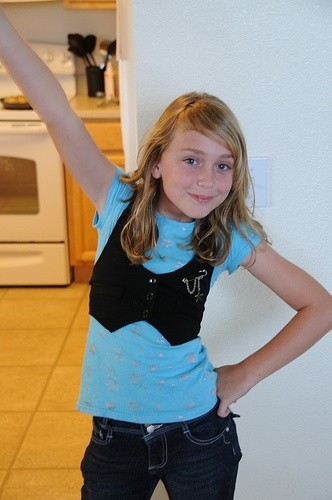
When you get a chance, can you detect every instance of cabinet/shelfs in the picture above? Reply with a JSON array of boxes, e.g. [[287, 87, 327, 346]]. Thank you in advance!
[[63, 117, 124, 282], [63, 0, 115, 10]]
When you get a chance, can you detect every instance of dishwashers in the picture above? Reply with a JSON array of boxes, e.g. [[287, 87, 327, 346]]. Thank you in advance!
[[0, 120, 72, 286]]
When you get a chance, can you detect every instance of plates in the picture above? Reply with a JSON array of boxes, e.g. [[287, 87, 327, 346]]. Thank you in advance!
[[0, 98, 33, 109]]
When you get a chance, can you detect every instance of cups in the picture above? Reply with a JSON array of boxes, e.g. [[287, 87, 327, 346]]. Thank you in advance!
[[86, 67, 105, 98]]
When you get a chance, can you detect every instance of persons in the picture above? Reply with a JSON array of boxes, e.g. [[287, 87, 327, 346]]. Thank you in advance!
[[0, 8, 332, 500]]
